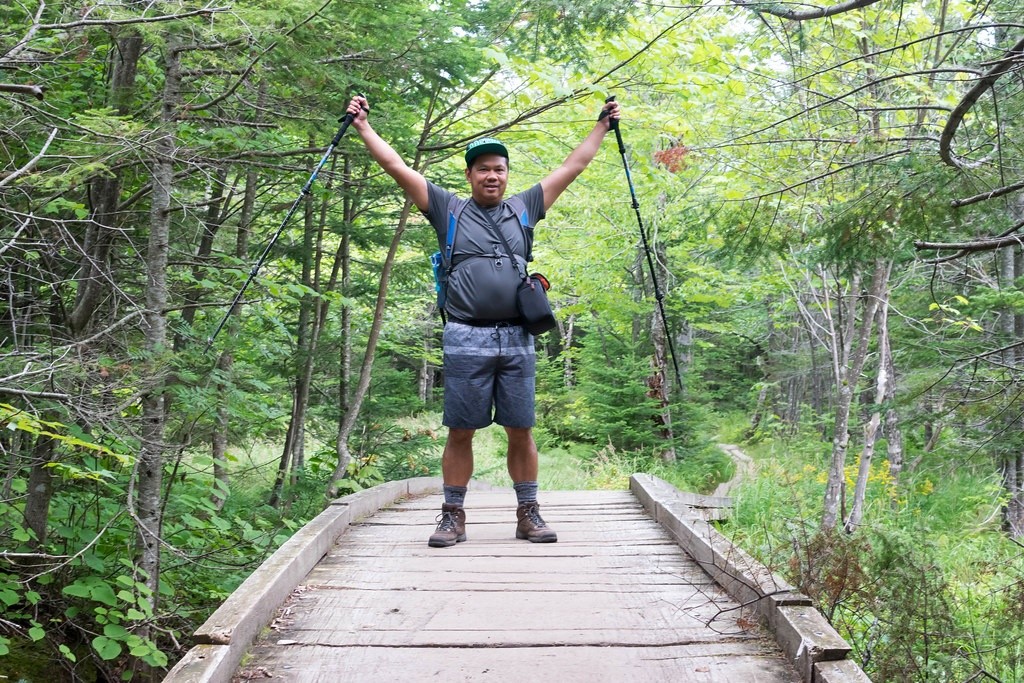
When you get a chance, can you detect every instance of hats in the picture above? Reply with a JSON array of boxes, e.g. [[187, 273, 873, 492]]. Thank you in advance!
[[465, 138, 510, 167]]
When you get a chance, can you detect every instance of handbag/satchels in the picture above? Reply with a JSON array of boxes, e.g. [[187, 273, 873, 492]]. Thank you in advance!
[[517, 273, 556, 337]]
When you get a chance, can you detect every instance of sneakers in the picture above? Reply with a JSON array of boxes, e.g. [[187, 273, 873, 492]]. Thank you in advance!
[[428, 502, 467, 545], [516, 502, 557, 541]]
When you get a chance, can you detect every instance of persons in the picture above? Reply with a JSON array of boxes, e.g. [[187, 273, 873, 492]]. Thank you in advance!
[[347, 95, 621, 548]]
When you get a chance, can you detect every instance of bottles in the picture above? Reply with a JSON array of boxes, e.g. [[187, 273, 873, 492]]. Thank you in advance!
[[430, 250, 443, 292]]
